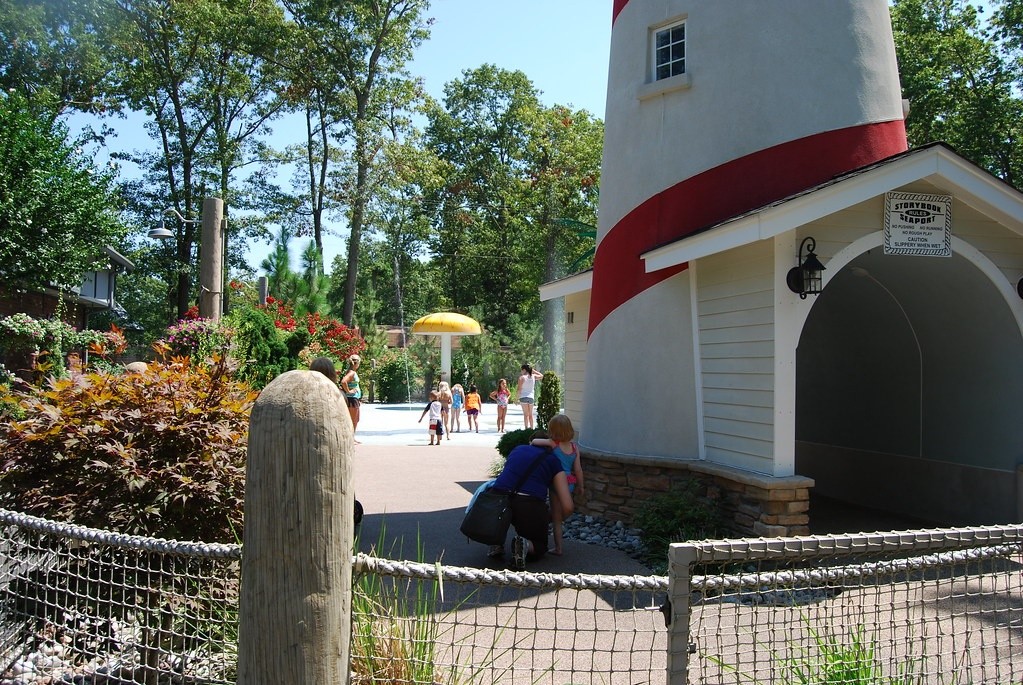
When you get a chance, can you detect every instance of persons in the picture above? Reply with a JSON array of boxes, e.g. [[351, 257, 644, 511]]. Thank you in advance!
[[487, 429, 575, 572], [309, 357, 348, 406], [463, 385, 483, 434], [529, 413, 584, 557], [340, 355, 363, 446], [437, 382, 453, 441], [489, 379, 512, 435], [514, 365, 544, 431], [418, 390, 447, 447], [450, 384, 467, 433]]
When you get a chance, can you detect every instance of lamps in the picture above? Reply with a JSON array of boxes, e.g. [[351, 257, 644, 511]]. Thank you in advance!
[[785, 237, 827, 301]]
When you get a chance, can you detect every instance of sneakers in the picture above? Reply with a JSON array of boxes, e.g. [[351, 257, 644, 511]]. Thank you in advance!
[[486, 544, 506, 559], [507, 534, 529, 572]]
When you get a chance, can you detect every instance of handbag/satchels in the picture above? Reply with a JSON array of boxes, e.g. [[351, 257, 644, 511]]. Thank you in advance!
[[460, 492, 513, 545]]
